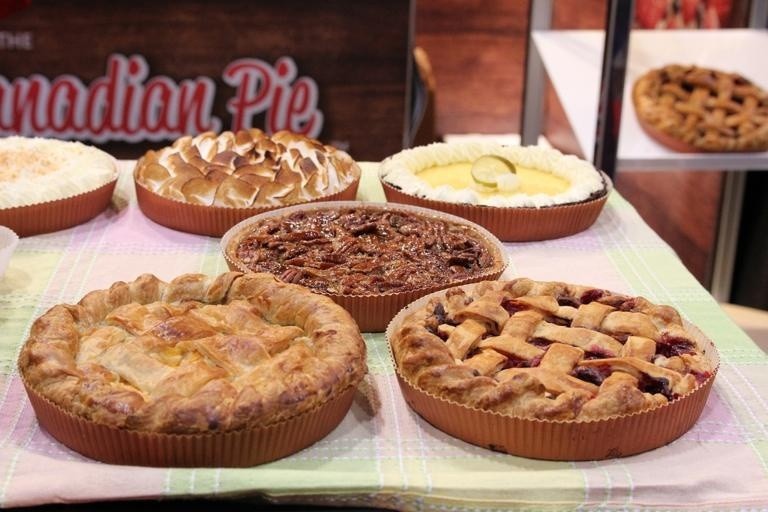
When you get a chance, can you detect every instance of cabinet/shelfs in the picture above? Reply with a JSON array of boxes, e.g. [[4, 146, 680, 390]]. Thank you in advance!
[[522, 0, 767, 355]]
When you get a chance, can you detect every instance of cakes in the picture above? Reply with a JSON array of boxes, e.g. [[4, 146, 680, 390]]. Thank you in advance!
[[20, 271, 368, 436], [138, 122, 357, 206], [632, 63, 768, 151], [391, 278, 712, 420], [227, 201, 500, 294], [384, 135, 607, 208], [0, 135, 116, 209]]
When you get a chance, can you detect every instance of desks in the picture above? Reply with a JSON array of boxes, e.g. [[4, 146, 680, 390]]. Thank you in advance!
[[1, 158, 767, 512]]
